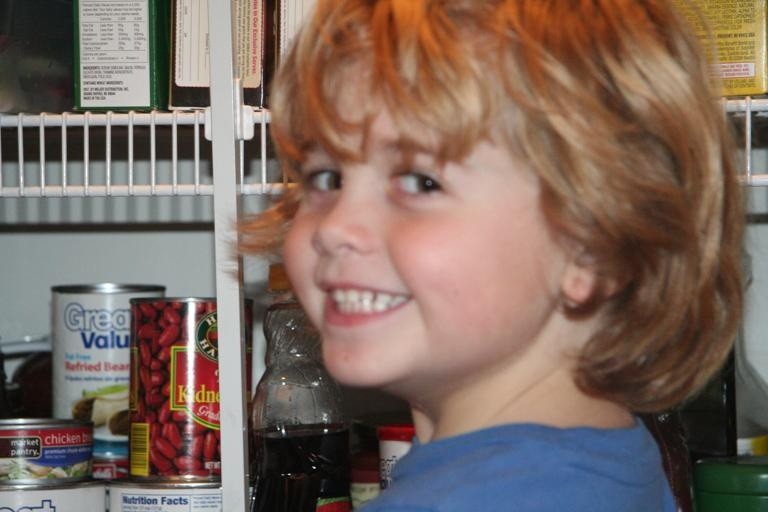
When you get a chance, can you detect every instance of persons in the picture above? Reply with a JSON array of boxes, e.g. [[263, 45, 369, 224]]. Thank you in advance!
[[220, 0, 750, 511]]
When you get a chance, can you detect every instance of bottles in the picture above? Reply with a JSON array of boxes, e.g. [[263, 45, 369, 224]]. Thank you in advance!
[[251, 261, 357, 512]]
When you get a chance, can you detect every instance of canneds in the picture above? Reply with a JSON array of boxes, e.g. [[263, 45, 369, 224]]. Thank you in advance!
[[127, 297, 222, 486], [0, 418, 94, 486], [51, 283, 166, 483], [0, 485, 108, 511], [109, 486, 222, 512]]
